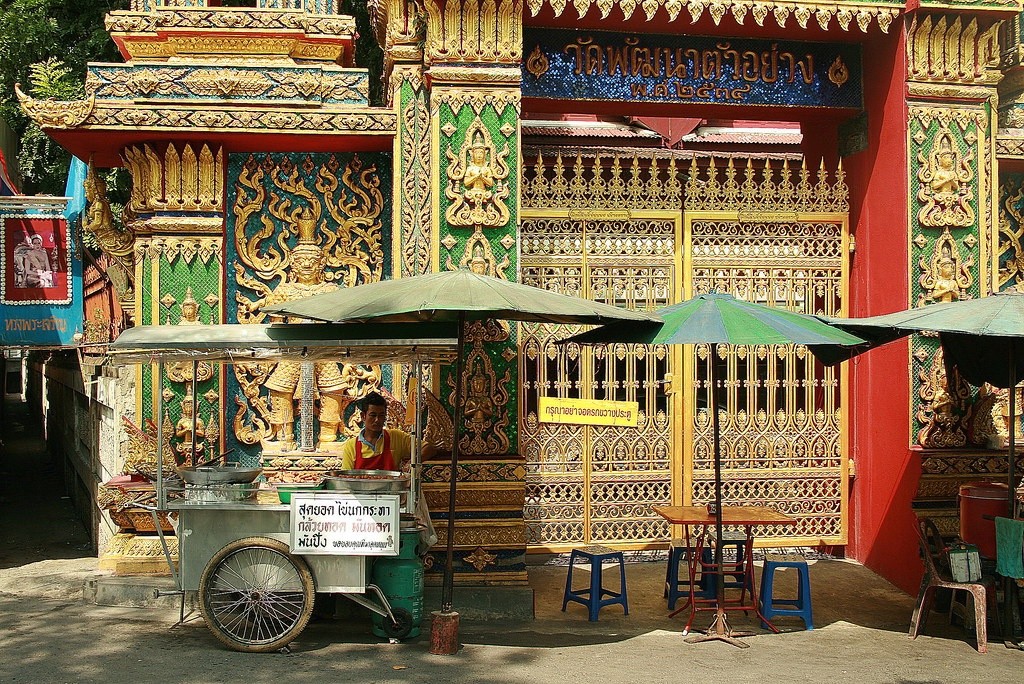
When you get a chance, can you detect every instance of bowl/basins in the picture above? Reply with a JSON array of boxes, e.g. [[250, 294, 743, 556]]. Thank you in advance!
[[271, 482, 326, 504]]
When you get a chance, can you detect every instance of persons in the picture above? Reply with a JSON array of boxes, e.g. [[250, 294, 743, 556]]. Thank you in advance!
[[933, 139, 959, 195], [260, 211, 347, 453], [469, 246, 488, 276], [934, 252, 959, 303], [463, 137, 494, 193], [176, 386, 205, 445], [174, 288, 204, 326], [342, 392, 433, 470], [464, 364, 494, 422]]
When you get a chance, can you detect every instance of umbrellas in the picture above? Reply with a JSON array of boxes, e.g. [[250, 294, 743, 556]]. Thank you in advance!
[[806, 281, 1024, 637], [552, 293, 866, 634], [260, 272, 665, 613]]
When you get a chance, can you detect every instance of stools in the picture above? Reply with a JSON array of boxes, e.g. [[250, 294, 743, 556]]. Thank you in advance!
[[663, 538, 717, 610], [758, 554, 813, 631], [701, 532, 753, 601], [561, 545, 629, 623]]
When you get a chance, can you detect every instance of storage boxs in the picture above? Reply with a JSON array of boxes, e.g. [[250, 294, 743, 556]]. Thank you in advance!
[[917, 533, 982, 583]]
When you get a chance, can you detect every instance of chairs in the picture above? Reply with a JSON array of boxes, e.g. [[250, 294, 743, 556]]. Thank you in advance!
[[908, 517, 1004, 653]]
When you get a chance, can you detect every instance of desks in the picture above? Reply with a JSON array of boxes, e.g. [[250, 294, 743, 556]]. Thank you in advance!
[[652, 505, 797, 634]]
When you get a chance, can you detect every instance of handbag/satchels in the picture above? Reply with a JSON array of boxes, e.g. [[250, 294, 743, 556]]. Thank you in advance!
[[949, 549, 982, 583]]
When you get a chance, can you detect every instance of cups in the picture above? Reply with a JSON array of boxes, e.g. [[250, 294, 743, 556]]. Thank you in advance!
[[707, 500, 718, 515]]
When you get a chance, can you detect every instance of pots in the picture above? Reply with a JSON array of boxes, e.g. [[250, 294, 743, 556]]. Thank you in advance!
[[176, 462, 263, 485], [320, 469, 412, 491]]
